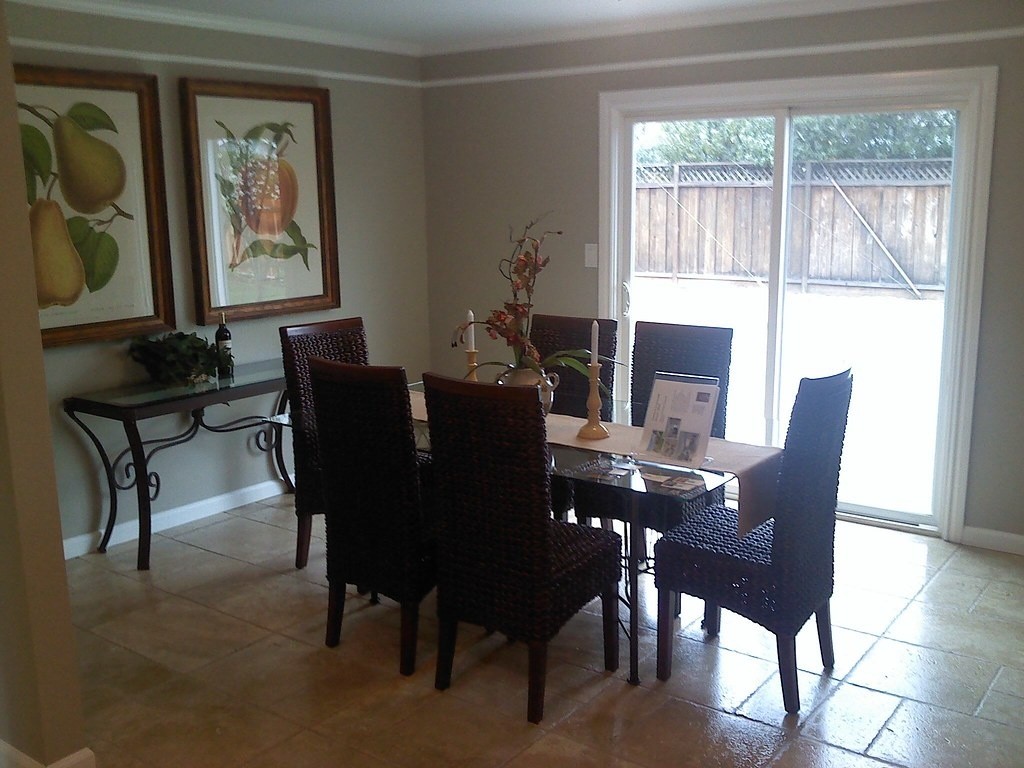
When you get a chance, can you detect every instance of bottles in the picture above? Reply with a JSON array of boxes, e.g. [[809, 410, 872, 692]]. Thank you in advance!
[[215, 312, 233, 379]]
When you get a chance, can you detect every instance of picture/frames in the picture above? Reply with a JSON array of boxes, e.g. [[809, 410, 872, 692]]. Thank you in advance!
[[13, 62, 177, 350], [176, 77, 341, 326]]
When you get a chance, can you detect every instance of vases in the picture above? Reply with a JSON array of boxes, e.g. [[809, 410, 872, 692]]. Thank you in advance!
[[495, 369, 560, 417]]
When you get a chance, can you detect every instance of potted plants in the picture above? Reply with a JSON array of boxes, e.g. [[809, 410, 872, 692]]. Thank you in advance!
[[126, 332, 235, 390]]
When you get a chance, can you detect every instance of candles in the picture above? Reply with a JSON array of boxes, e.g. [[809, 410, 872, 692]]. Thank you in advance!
[[467, 309, 475, 350], [591, 319, 598, 365]]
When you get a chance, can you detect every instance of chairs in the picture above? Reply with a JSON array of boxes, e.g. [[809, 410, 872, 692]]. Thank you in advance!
[[654, 368, 855, 716], [279, 317, 372, 570], [422, 371, 622, 725], [574, 321, 735, 619], [306, 355, 437, 678], [530, 314, 618, 531]]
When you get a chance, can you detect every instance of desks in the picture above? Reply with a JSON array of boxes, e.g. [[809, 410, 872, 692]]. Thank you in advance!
[[62, 357, 294, 571], [262, 382, 783, 687]]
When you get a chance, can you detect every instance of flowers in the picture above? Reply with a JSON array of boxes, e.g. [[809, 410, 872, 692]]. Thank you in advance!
[[451, 209, 627, 398]]
